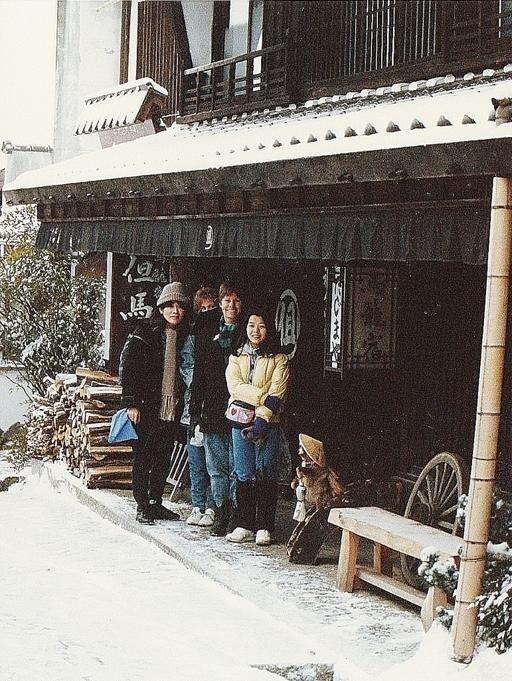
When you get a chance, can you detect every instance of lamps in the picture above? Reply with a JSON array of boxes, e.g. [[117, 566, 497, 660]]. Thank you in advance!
[[210, 183, 222, 192], [288, 176, 303, 187], [336, 172, 354, 183], [6, 186, 167, 206], [386, 167, 408, 179], [182, 184, 191, 194], [248, 180, 259, 191], [442, 164, 460, 177]]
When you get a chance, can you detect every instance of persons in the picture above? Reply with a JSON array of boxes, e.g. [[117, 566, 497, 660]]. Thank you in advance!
[[287, 432, 345, 530], [222, 303, 288, 549], [119, 280, 191, 526], [180, 282, 224, 530], [192, 281, 253, 538]]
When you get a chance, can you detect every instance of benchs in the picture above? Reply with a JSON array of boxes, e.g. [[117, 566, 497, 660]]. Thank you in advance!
[[326, 505, 469, 611]]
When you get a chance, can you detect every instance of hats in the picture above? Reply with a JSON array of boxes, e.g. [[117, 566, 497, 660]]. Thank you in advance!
[[298, 433, 326, 468], [154, 280, 188, 307]]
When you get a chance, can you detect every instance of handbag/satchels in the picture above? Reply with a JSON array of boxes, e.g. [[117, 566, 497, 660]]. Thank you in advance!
[[224, 400, 254, 433]]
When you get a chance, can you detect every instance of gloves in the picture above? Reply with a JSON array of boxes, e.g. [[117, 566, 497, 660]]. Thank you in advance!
[[252, 417, 267, 439]]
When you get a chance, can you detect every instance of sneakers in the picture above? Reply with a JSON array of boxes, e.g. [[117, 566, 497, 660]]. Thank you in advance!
[[151, 503, 181, 521], [225, 527, 254, 545], [254, 529, 272, 545], [185, 507, 202, 526], [198, 508, 216, 527], [135, 506, 156, 527]]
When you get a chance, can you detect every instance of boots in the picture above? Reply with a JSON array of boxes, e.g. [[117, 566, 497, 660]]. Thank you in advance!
[[209, 498, 230, 538]]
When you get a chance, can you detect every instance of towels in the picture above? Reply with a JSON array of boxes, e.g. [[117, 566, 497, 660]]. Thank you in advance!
[[108, 408, 139, 443]]
